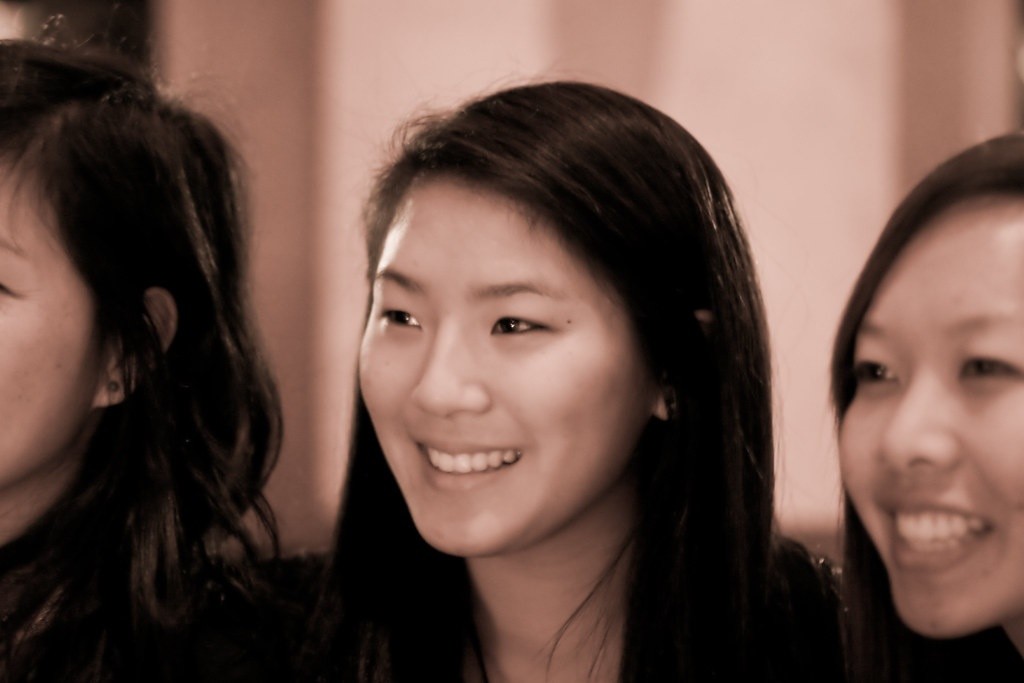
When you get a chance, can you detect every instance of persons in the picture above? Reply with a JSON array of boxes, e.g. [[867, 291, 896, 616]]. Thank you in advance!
[[0, 38, 283, 683], [242, 79, 848, 683], [827, 133, 1024, 683]]
